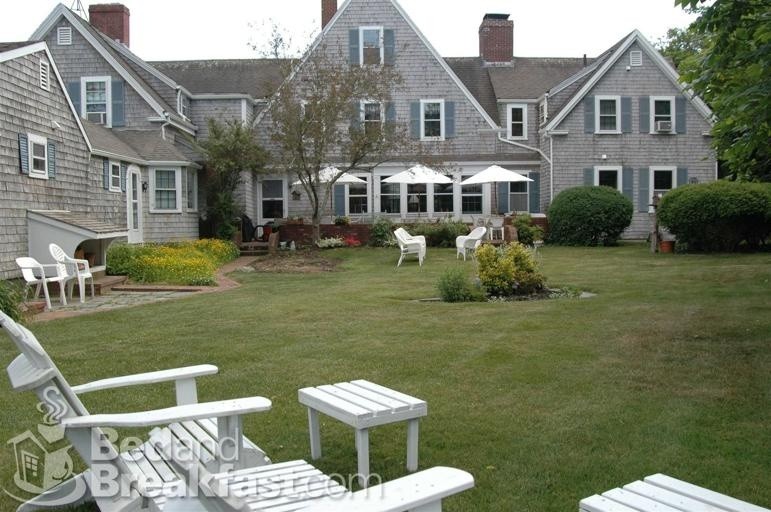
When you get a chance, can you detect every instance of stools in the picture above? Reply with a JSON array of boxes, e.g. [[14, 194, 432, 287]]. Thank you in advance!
[[579, 470, 770, 512], [297, 378, 428, 488]]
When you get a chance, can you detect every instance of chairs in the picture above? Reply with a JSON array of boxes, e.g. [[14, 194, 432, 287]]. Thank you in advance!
[[393, 230, 422, 266], [454, 226, 486, 263], [486, 217, 505, 241], [15, 257, 67, 310], [0, 309, 272, 512], [471, 215, 476, 229], [49, 243, 95, 302], [397, 226, 426, 258], [146, 425, 480, 511]]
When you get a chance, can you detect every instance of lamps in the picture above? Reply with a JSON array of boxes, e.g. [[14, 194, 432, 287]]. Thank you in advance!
[[142, 181, 149, 192]]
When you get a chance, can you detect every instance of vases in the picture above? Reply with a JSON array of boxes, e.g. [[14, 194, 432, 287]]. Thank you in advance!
[[659, 240, 676, 253]]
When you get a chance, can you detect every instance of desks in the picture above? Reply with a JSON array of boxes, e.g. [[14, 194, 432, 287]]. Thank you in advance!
[[479, 217, 492, 240]]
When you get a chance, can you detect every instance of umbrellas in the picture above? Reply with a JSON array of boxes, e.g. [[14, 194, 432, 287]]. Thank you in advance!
[[291, 164, 369, 225], [380, 164, 458, 219], [458, 164, 537, 239]]
